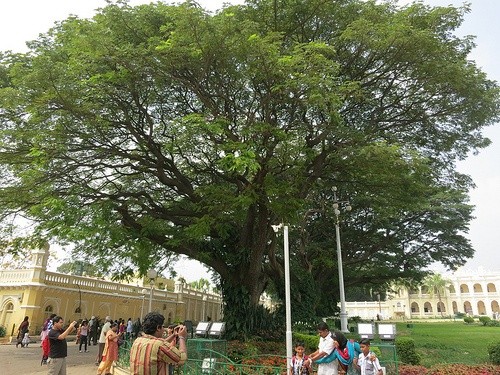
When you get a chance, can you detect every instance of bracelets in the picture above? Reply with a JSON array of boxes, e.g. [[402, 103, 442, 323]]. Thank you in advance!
[[308, 357, 312, 368], [178, 336, 185, 341]]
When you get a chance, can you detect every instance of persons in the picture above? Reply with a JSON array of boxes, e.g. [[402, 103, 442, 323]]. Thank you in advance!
[[353, 339, 383, 375], [76, 316, 143, 366], [303, 322, 338, 375], [97, 320, 122, 375], [15, 316, 31, 348], [48, 316, 77, 375], [40, 314, 57, 366], [130, 311, 188, 375], [289, 343, 312, 375], [313, 332, 376, 375]]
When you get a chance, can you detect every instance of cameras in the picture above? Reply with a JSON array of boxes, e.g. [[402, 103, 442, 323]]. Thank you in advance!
[[74, 322, 79, 327], [177, 327, 183, 332]]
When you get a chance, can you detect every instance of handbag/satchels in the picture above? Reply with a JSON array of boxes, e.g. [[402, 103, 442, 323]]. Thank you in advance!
[[373, 366, 386, 375], [118, 335, 123, 346]]
